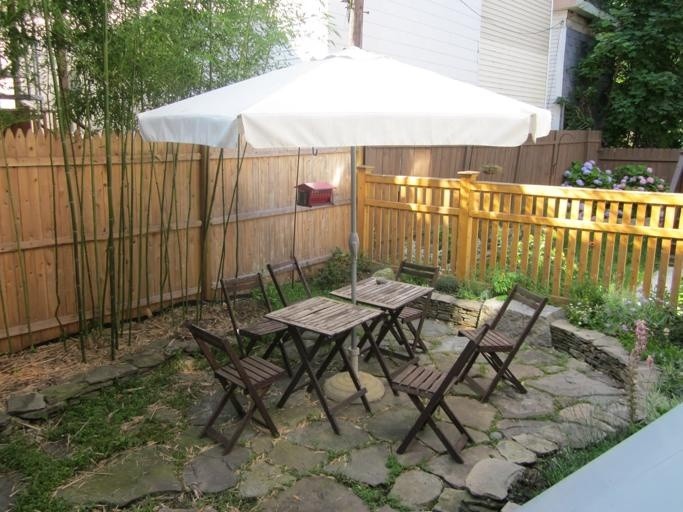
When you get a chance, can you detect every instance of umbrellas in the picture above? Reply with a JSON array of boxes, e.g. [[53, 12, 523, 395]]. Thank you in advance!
[[136, 45, 551, 377]]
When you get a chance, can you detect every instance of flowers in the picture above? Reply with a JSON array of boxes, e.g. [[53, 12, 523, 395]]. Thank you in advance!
[[561, 159, 669, 190]]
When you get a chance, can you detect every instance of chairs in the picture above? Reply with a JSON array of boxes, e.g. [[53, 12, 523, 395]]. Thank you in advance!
[[389, 322, 490, 464], [458, 282, 548, 403], [217, 256, 439, 434], [184, 317, 289, 456]]
